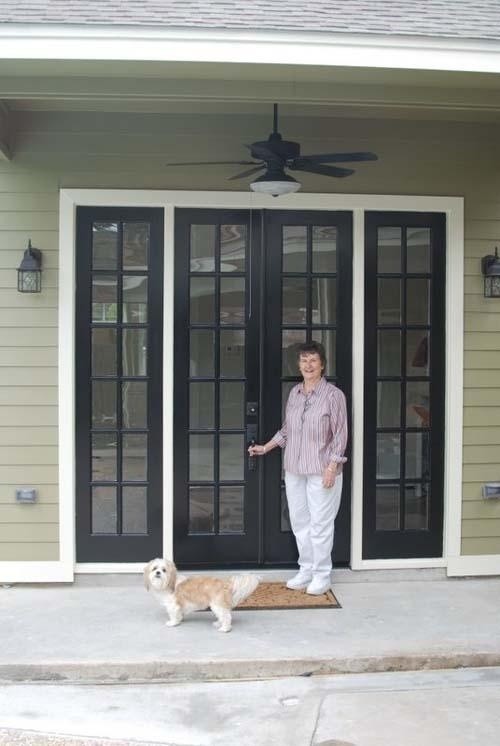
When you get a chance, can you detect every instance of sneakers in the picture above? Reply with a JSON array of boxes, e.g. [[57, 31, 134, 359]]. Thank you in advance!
[[307, 581, 331, 595], [286, 575, 312, 590]]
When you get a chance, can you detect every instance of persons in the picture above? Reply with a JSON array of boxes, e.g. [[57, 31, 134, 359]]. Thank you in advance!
[[248, 342, 348, 596]]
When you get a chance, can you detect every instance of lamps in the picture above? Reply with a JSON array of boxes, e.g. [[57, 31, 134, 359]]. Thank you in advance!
[[480, 247, 500, 298], [16, 239, 42, 293], [249, 172, 300, 198]]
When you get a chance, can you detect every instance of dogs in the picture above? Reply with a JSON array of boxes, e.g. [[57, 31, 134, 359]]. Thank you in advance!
[[143, 558, 260, 633]]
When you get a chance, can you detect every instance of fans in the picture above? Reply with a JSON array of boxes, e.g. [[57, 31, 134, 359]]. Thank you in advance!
[[166, 104, 377, 180]]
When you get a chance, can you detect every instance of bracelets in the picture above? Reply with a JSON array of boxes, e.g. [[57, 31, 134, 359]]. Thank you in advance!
[[263, 446, 266, 455], [328, 468, 336, 473]]
